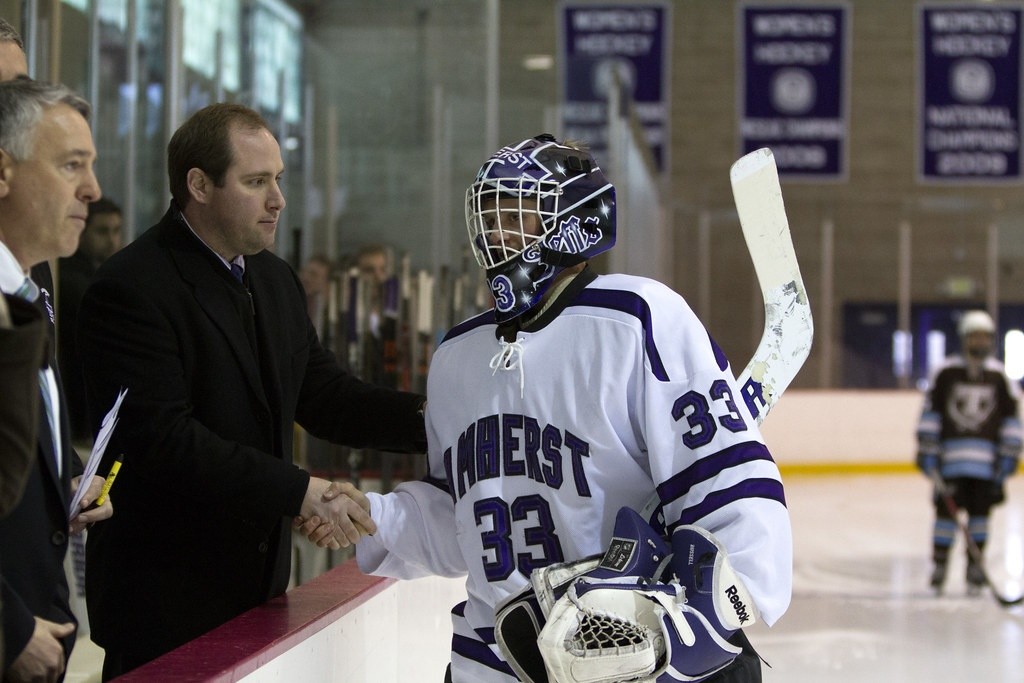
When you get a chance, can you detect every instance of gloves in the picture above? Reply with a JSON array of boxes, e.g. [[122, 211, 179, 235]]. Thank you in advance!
[[533, 505, 759, 680]]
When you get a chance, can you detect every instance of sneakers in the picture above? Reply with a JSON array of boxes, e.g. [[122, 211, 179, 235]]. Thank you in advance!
[[964, 561, 989, 597], [929, 562, 948, 596]]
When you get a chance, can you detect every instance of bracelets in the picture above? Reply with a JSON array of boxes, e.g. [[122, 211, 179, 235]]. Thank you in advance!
[[417, 399, 425, 429]]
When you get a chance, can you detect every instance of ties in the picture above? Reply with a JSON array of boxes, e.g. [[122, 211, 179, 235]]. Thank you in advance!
[[229, 265, 245, 286]]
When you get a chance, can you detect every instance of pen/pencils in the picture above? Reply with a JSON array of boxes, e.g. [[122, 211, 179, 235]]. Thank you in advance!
[[85, 453, 125, 532]]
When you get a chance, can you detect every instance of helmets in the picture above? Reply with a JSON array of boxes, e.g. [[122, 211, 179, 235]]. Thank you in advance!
[[959, 310, 999, 355], [463, 131, 620, 319]]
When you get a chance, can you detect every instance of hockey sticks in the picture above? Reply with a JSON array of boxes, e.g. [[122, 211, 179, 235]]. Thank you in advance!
[[928, 466, 1024, 609], [530, 145, 815, 622]]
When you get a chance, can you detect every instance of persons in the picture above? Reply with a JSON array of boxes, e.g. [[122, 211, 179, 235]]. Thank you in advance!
[[288, 132, 794, 683], [30, 198, 392, 352], [914, 309, 1023, 596], [0, 75, 113, 683], [75, 103, 430, 683]]
